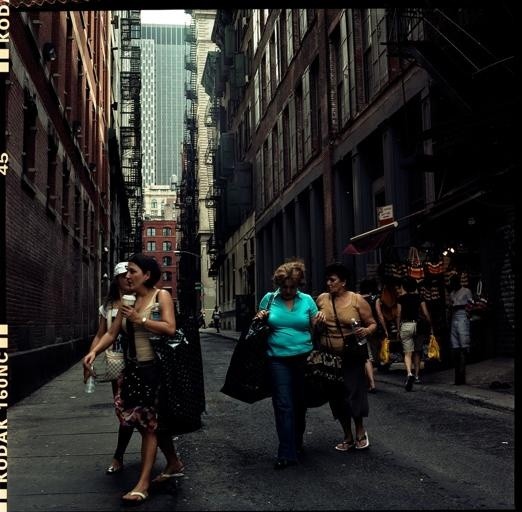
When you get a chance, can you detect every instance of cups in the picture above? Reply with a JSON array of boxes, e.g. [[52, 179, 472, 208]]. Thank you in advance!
[[121, 294, 136, 318]]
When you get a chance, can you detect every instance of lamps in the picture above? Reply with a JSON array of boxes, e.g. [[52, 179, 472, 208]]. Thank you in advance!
[[41, 41, 97, 174]]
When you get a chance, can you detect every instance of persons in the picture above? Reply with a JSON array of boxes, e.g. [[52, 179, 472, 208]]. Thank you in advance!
[[212, 306, 222, 333], [84, 261, 137, 474], [83, 253, 185, 503]]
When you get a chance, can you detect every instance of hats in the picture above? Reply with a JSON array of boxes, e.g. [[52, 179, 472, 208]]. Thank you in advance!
[[112, 260, 132, 277]]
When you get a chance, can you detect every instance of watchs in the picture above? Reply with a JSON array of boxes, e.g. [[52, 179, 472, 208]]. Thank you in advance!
[[141, 317, 147, 327]]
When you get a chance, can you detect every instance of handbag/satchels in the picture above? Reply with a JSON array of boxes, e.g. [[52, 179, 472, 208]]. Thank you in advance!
[[292, 349, 344, 408], [337, 332, 370, 373], [149, 320, 210, 438], [91, 348, 125, 384], [379, 339, 394, 366], [217, 320, 281, 406], [421, 333, 443, 364], [382, 247, 469, 302]]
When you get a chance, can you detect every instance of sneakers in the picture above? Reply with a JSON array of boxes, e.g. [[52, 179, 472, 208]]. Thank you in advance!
[[274, 448, 302, 467], [105, 463, 125, 475], [405, 374, 414, 393], [413, 375, 421, 384]]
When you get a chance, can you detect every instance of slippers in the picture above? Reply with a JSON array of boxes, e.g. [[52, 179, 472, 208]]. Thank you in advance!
[[152, 470, 191, 484], [118, 489, 152, 507], [334, 438, 357, 451], [354, 429, 370, 449]]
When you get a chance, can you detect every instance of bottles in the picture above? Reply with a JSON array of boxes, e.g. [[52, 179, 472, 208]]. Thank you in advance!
[[151, 302, 162, 340], [350, 316, 367, 345], [85, 362, 95, 393]]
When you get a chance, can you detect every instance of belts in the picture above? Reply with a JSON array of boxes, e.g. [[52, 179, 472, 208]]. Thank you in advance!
[[401, 320, 419, 323]]
